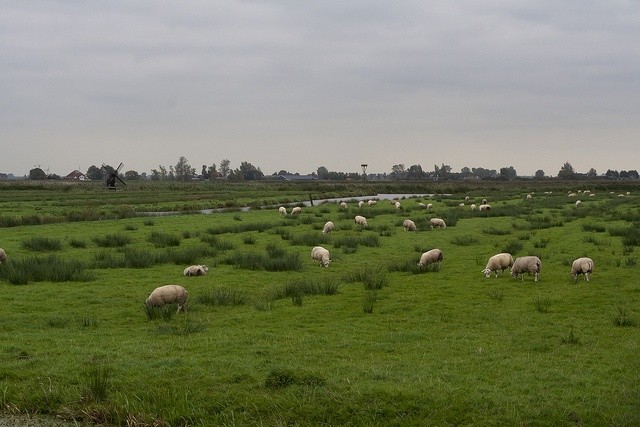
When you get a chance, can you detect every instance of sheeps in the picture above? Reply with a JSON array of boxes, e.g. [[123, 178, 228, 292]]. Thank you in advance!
[[403, 220, 417, 232], [626, 192, 631, 195], [479, 205, 485, 211], [321, 221, 335, 236], [485, 205, 491, 210], [471, 204, 476, 211], [544, 191, 549, 194], [568, 191, 571, 193], [576, 200, 581, 206], [355, 216, 367, 228], [568, 193, 575, 197], [279, 207, 287, 215], [510, 256, 542, 282], [583, 190, 590, 194], [341, 202, 347, 208], [429, 218, 447, 231], [370, 201, 377, 205], [368, 199, 372, 206], [549, 191, 553, 194], [481, 253, 515, 278], [188, 266, 206, 276], [419, 203, 426, 208], [311, 246, 332, 268], [465, 195, 470, 201], [460, 203, 465, 207], [570, 258, 594, 282], [578, 190, 582, 193], [618, 194, 624, 197], [146, 285, 188, 315], [589, 193, 595, 197], [610, 192, 614, 194], [395, 201, 401, 209], [427, 204, 433, 209], [416, 249, 443, 269], [482, 199, 487, 204], [184, 264, 208, 277], [358, 201, 364, 208], [291, 207, 302, 215], [527, 195, 532, 199]]
